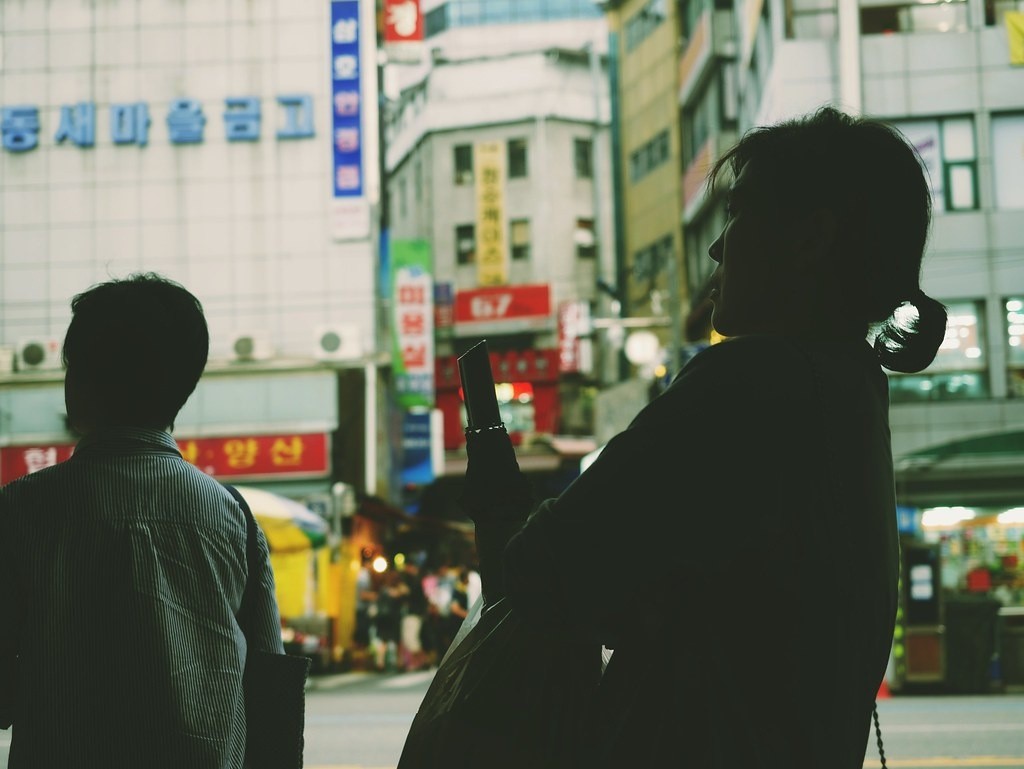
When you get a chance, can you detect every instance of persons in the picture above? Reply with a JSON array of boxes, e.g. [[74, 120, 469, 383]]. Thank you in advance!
[[353, 546, 482, 672], [396, 104, 947, 769], [0, 272, 285, 769]]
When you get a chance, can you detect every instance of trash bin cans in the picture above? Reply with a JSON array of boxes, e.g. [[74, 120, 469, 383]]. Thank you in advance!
[[942, 590, 1003, 695]]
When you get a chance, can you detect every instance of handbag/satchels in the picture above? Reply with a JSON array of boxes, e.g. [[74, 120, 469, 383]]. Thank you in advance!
[[224, 483, 312, 769], [398, 585, 603, 769]]
